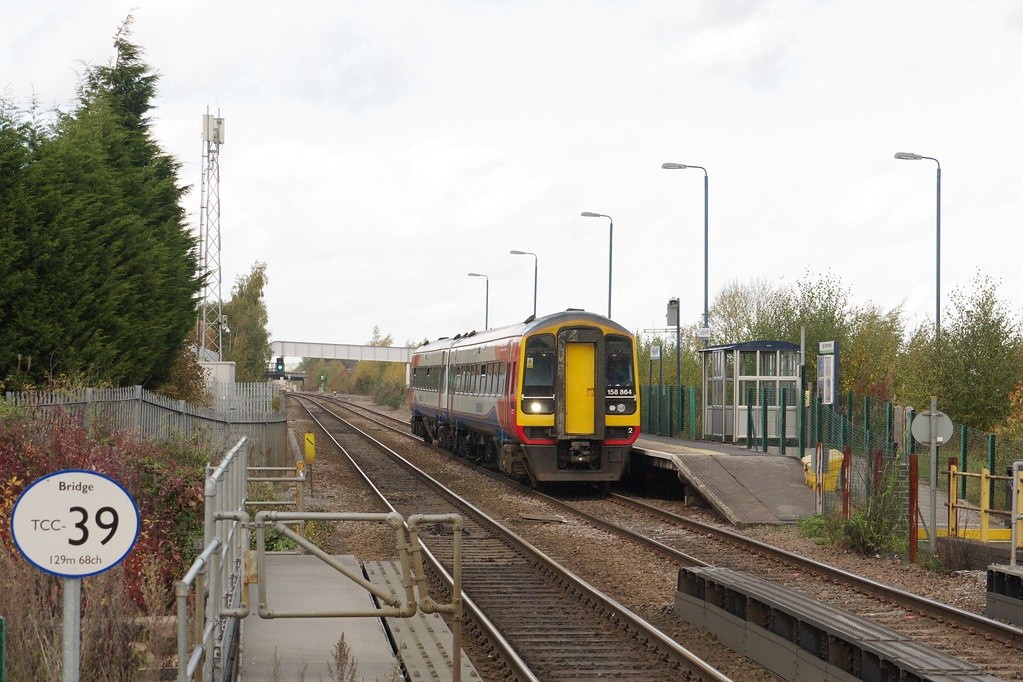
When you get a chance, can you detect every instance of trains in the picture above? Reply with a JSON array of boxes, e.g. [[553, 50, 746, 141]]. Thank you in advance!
[[406, 309, 644, 492]]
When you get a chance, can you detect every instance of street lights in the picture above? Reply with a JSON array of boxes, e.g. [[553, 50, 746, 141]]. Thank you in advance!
[[468, 272, 489, 331], [582, 211, 613, 320], [896, 152, 945, 406], [661, 161, 710, 347], [510, 250, 538, 318]]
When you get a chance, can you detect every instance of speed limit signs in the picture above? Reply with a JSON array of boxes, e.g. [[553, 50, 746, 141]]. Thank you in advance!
[[10, 471, 137, 576]]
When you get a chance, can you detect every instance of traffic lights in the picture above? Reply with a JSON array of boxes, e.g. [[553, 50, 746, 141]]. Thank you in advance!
[[275, 356, 284, 376]]
